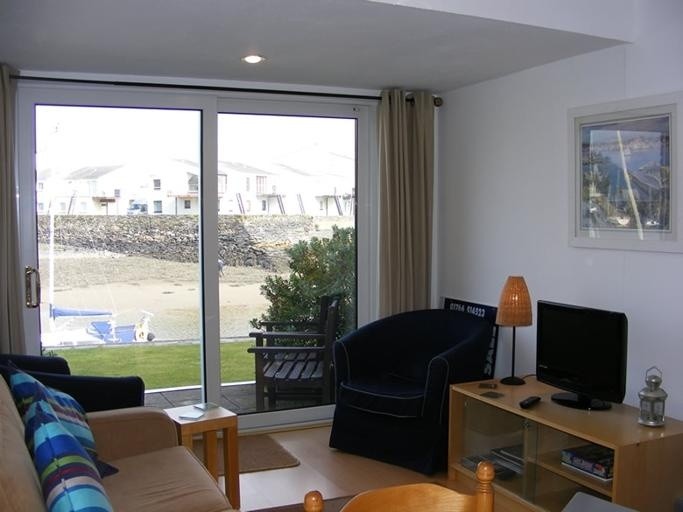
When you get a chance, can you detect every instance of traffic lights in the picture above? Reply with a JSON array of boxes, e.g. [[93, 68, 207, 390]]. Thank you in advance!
[[8, 358, 113, 512]]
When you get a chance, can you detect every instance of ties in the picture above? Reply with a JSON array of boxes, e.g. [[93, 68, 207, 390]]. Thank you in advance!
[[535, 299, 629, 411]]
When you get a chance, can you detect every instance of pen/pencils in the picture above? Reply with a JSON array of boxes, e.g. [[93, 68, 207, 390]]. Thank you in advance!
[[128, 201, 148, 216]]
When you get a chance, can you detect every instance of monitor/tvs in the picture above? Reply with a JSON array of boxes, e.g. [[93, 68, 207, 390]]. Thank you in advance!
[[460, 443, 524, 482], [559, 461, 613, 482]]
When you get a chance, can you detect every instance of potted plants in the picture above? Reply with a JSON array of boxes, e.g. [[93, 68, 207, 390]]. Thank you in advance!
[[566, 88, 682, 256]]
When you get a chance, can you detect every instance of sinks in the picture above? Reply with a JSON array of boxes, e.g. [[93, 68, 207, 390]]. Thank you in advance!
[[0, 377, 232, 511]]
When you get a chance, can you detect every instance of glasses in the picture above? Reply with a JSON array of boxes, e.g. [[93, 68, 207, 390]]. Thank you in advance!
[[496, 274, 534, 387]]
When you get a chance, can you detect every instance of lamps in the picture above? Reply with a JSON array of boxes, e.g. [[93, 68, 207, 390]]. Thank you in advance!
[[0, 352, 143, 409], [332, 307, 493, 476], [249, 292, 341, 412]]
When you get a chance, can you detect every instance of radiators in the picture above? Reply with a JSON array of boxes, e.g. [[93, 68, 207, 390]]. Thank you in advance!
[[519, 396, 541, 409]]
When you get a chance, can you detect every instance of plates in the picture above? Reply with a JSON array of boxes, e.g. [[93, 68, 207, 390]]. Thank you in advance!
[[445, 379, 682, 511]]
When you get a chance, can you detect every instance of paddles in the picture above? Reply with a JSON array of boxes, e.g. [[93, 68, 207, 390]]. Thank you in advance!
[[191, 432, 300, 476]]
